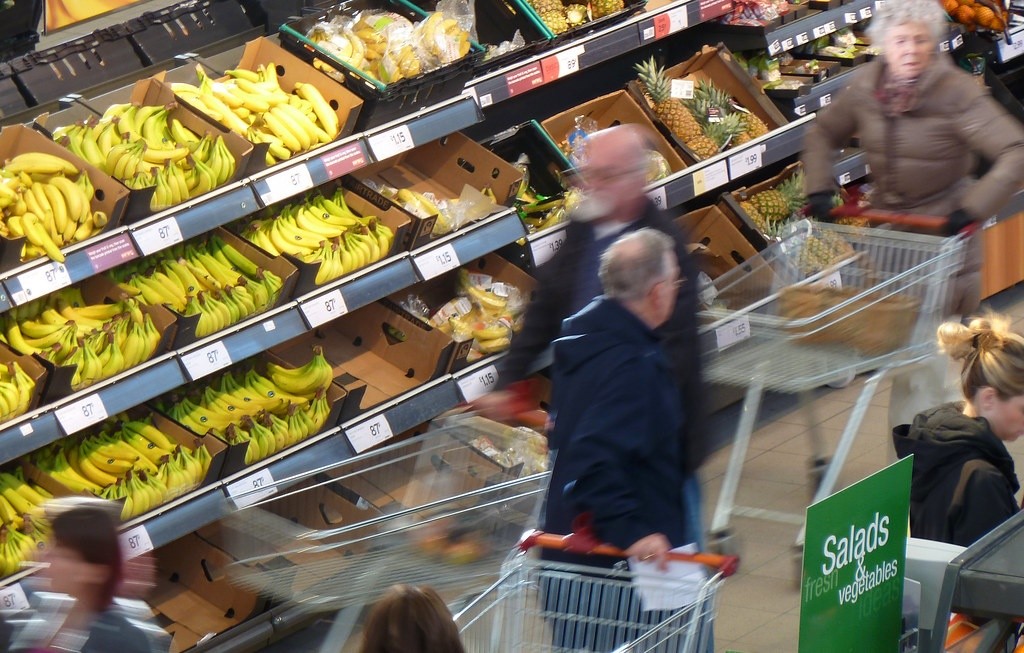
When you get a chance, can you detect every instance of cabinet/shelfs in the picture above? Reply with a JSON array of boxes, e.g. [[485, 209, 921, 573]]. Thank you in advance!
[[463, 0, 821, 412], [0, 96, 569, 653], [683, 0, 1024, 296]]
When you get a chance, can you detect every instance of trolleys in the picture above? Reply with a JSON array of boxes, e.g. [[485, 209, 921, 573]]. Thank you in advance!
[[217, 377, 571, 653], [689, 200, 987, 589], [449, 528, 742, 653]]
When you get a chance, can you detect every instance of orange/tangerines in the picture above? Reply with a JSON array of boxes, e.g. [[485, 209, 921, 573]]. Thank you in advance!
[[940, 0, 1009, 32]]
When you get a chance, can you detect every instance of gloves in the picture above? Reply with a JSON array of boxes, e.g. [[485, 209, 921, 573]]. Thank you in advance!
[[945, 209, 972, 234], [810, 193, 837, 222]]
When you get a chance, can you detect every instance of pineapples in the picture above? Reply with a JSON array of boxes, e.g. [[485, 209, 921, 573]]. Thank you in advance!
[[633, 55, 769, 160], [509, 0, 624, 34], [740, 168, 871, 278]]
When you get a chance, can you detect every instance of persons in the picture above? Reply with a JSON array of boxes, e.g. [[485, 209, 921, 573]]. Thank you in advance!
[[474, 126, 702, 553], [807, 0, 1024, 423], [359, 585, 464, 653], [0, 506, 149, 653], [533, 229, 715, 651], [893, 317, 1024, 550]]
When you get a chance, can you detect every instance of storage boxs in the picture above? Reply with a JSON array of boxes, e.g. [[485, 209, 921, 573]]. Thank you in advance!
[[0, 0, 1024, 653]]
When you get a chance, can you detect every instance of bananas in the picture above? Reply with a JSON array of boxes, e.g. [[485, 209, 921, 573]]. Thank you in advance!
[[0, 12, 576, 581]]
